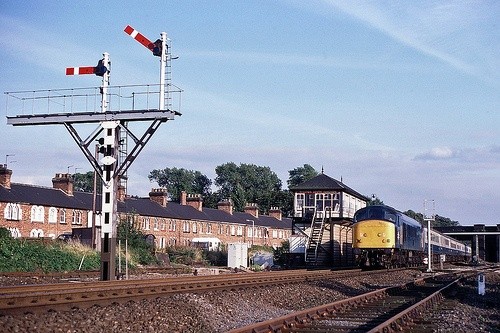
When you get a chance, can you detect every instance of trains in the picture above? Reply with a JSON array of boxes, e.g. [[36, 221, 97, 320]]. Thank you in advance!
[[345, 205, 472, 269]]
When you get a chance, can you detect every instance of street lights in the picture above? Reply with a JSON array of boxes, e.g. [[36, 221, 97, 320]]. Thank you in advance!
[[422, 199, 436, 273], [246, 219, 255, 245]]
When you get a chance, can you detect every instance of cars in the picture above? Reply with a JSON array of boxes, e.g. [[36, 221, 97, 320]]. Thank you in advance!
[[56, 232, 71, 242]]
